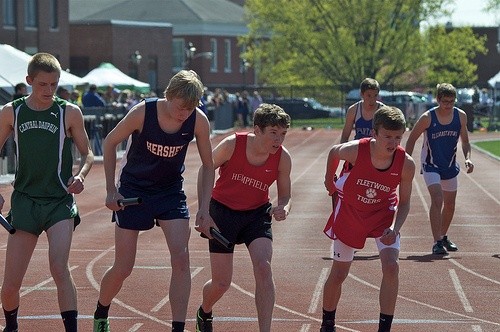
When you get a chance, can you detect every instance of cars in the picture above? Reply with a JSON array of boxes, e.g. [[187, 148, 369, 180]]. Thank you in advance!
[[261, 87, 495, 121]]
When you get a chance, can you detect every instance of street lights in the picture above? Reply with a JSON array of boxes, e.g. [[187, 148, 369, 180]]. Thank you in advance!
[[131, 50, 142, 81], [239, 58, 251, 129], [184, 41, 196, 69]]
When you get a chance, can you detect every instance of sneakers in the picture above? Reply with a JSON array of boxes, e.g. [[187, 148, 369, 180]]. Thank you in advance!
[[93, 310, 110, 332], [196, 306, 213, 332], [441, 236, 457, 250], [433, 240, 446, 254]]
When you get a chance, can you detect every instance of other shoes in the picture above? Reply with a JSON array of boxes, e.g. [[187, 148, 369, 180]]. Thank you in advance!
[[378, 323, 391, 332], [320, 320, 336, 332], [2, 322, 18, 332]]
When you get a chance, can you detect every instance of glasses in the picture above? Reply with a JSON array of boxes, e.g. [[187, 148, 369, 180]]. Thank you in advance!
[[440, 99, 454, 105]]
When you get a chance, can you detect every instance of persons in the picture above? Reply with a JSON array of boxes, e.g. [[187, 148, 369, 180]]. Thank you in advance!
[[319, 107, 416, 332], [405, 83, 474, 255], [94, 69, 215, 332], [340, 78, 388, 144], [0, 53, 95, 332], [421, 88, 488, 109], [196, 103, 293, 332], [12, 83, 264, 156]]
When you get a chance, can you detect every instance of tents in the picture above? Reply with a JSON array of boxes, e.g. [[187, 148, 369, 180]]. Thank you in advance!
[[82, 62, 150, 93], [0, 43, 89, 95]]
